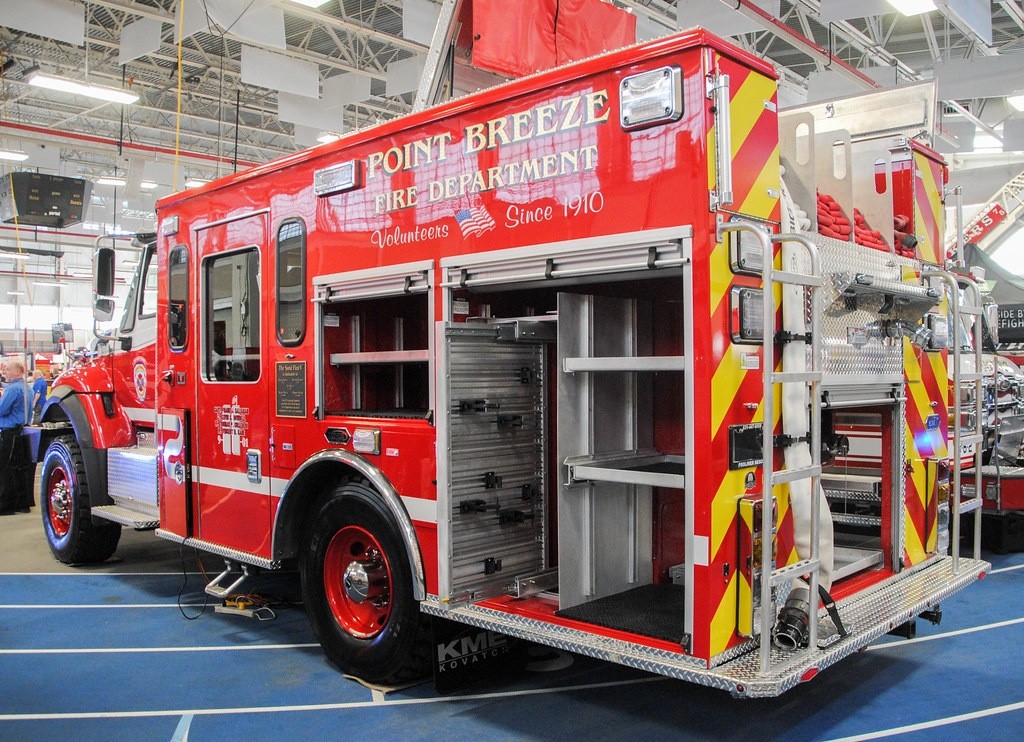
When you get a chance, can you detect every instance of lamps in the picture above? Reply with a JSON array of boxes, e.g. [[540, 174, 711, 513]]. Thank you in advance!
[[27, 71, 141, 106], [317, 131, 341, 143], [0, 251, 67, 296], [0, 149, 29, 161], [80, 175, 159, 188], [184, 176, 212, 188]]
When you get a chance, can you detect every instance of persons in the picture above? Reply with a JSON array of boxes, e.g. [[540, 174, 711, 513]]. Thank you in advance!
[[32, 369, 47, 425], [0, 362, 34, 515]]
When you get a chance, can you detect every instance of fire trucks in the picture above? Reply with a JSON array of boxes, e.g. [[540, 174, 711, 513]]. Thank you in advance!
[[31, 24, 997, 686], [818, 349, 1024, 555]]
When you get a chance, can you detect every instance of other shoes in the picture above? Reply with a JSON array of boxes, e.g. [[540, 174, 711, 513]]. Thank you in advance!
[[0, 508, 15, 515], [15, 505, 31, 513]]
